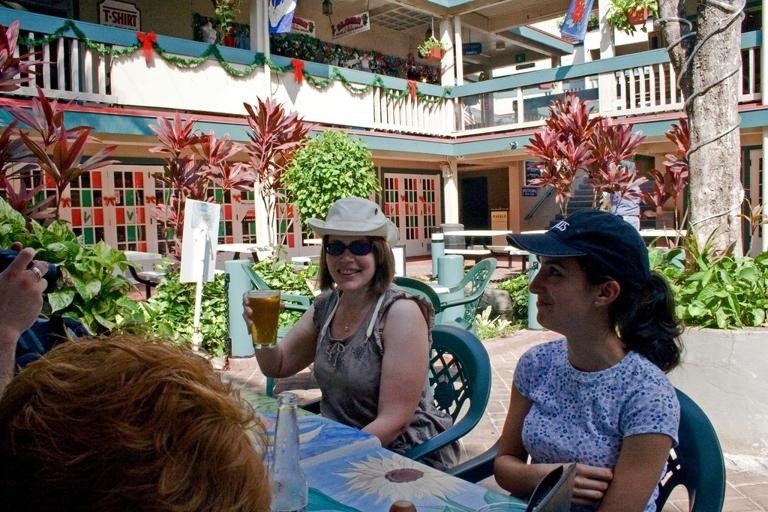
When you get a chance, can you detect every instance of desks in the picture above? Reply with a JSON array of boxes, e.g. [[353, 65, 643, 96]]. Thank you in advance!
[[122, 250, 161, 261], [637, 230, 688, 238], [444, 231, 512, 246], [520, 230, 550, 235], [218, 243, 270, 264], [304, 239, 324, 245], [239, 389, 383, 470], [258, 447, 538, 512]]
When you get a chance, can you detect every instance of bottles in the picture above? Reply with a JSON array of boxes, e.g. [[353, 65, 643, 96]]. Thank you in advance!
[[267, 391, 307, 512]]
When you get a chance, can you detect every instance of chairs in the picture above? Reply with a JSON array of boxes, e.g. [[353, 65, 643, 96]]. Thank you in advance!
[[240, 262, 312, 340], [392, 276, 441, 324], [444, 386, 726, 511], [431, 257, 497, 331], [128, 264, 165, 299], [295, 326, 492, 461]]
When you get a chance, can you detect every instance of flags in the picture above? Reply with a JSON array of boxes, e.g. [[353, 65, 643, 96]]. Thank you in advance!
[[265, 0, 296, 34]]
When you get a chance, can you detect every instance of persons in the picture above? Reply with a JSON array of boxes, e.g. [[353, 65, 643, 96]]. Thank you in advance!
[[493, 208, 685, 512], [0, 330, 269, 512], [241, 196, 463, 470], [0, 240, 50, 398]]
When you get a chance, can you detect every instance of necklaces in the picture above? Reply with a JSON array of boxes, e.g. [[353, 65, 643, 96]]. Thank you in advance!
[[341, 300, 367, 336]]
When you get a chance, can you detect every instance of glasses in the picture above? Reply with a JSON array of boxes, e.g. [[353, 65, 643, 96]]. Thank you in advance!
[[324, 240, 377, 256]]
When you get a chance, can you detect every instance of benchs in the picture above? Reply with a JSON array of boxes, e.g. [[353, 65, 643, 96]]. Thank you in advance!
[[291, 256, 313, 266], [509, 251, 540, 272], [444, 249, 492, 264], [467, 244, 517, 267]]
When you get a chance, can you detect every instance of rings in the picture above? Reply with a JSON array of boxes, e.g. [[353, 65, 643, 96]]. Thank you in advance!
[[32, 266, 43, 280]]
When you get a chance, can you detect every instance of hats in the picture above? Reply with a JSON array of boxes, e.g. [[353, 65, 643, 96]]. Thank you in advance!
[[506, 208, 649, 291], [308, 198, 400, 248]]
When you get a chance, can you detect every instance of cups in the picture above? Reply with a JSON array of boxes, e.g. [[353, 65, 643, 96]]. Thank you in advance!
[[244, 289, 280, 350]]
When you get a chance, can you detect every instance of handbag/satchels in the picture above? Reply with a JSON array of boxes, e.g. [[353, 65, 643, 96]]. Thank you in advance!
[[16, 304, 93, 368]]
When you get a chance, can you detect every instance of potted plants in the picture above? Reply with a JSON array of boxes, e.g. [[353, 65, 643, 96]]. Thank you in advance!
[[602, 0, 664, 36], [418, 36, 450, 61], [213, 0, 242, 46]]
